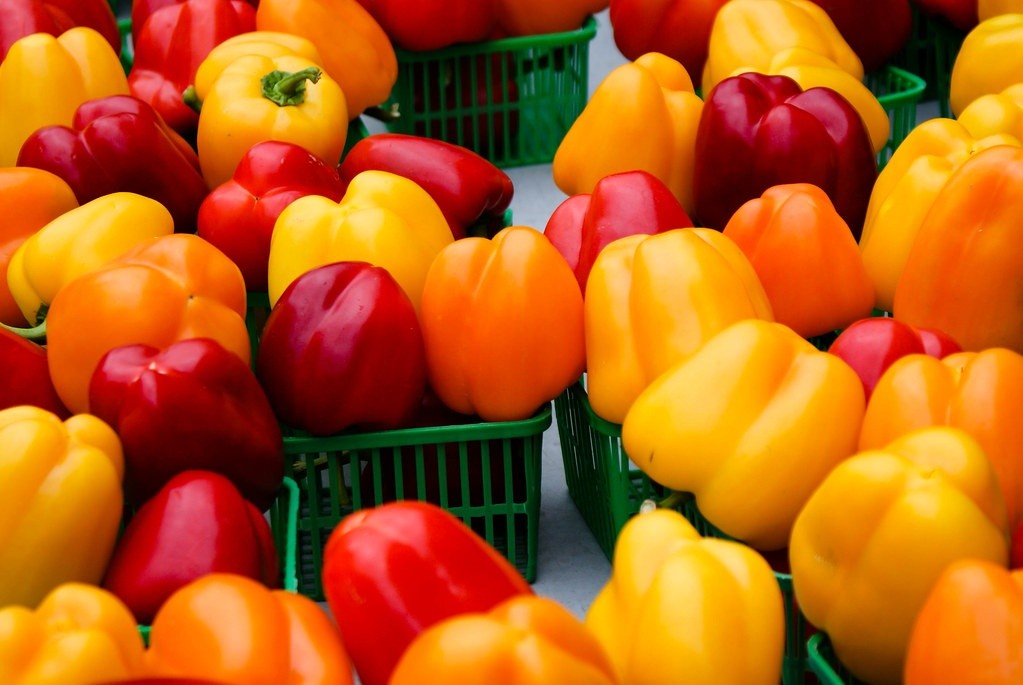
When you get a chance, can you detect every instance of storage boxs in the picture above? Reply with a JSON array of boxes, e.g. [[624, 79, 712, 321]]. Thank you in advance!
[[2, 1, 927, 684]]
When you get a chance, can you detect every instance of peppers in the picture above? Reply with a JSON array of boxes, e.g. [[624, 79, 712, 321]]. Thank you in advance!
[[0, 0, 1023, 685]]
